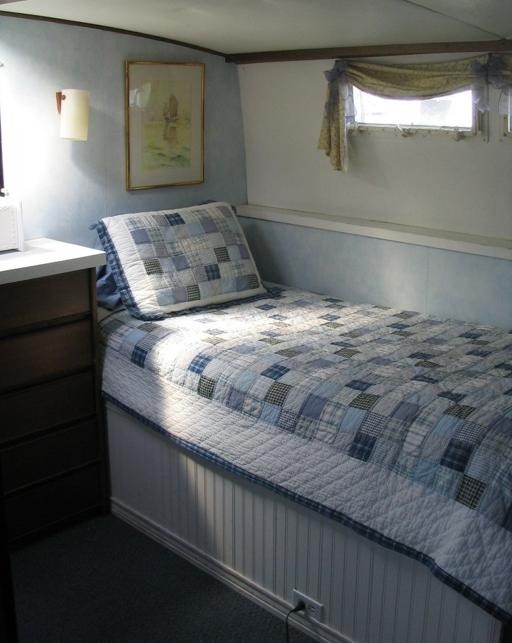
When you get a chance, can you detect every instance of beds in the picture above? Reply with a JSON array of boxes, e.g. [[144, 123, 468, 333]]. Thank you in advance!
[[99, 284, 512, 568]]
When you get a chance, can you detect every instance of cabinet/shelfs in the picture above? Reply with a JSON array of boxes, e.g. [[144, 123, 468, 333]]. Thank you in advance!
[[0, 268, 110, 555]]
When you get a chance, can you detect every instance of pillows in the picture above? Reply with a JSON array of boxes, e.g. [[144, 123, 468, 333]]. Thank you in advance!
[[94, 196, 270, 323]]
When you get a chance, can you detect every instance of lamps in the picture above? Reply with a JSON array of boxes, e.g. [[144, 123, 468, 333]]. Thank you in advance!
[[55, 88, 91, 143]]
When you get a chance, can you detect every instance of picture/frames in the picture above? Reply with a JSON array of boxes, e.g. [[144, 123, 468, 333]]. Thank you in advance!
[[123, 57, 208, 193]]
